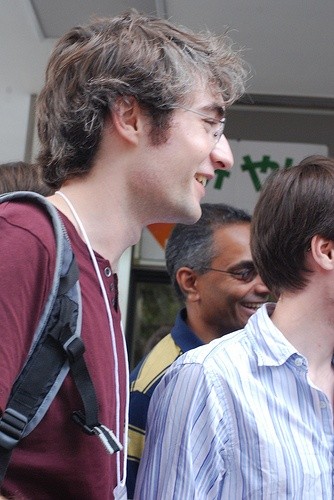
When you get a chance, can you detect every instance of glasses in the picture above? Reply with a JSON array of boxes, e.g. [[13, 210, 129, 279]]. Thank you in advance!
[[177, 105, 226, 137], [200, 268, 257, 283]]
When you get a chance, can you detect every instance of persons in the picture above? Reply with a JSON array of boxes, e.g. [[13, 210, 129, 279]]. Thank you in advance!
[[0, 7, 334, 500]]
